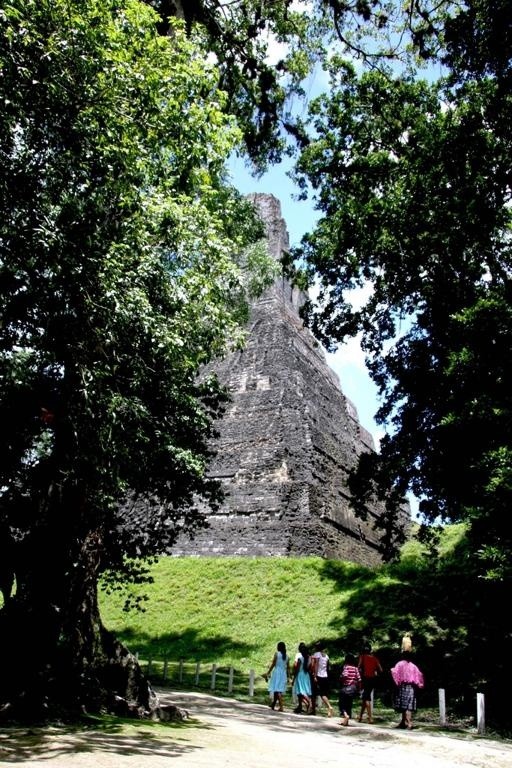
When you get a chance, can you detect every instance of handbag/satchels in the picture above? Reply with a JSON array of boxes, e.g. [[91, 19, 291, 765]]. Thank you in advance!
[[341, 686, 356, 698]]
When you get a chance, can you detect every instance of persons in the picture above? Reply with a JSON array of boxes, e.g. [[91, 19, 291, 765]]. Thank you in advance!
[[336, 651, 363, 725], [294, 640, 311, 708], [388, 648, 426, 728], [294, 646, 317, 715], [307, 639, 333, 718], [357, 640, 384, 723], [261, 641, 292, 711]]
